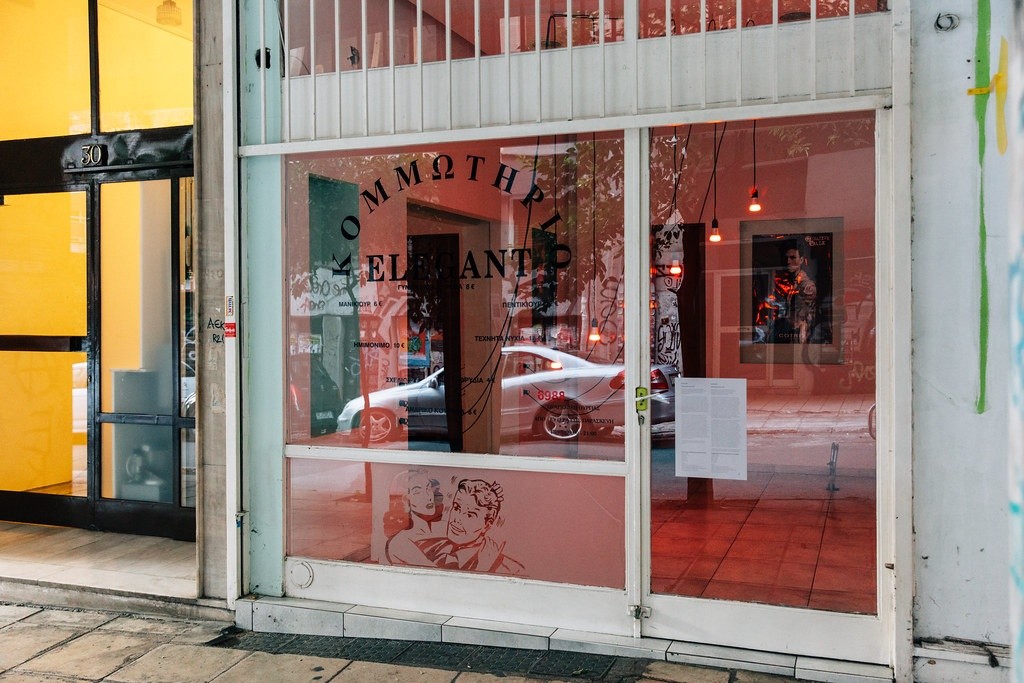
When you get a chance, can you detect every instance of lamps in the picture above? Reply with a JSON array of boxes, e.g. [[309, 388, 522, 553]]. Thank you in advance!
[[709, 123, 722, 242], [670, 126, 681, 275], [589, 131, 601, 341], [749, 120, 761, 211]]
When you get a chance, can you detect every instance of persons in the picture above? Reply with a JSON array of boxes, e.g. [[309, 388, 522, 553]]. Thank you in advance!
[[767, 244, 816, 344]]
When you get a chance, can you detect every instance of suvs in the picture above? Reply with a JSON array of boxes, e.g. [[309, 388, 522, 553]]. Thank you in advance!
[[180, 352, 342, 439]]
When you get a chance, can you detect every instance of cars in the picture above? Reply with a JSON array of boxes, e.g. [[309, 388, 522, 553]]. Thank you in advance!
[[332, 345, 678, 439], [125, 440, 196, 485]]
